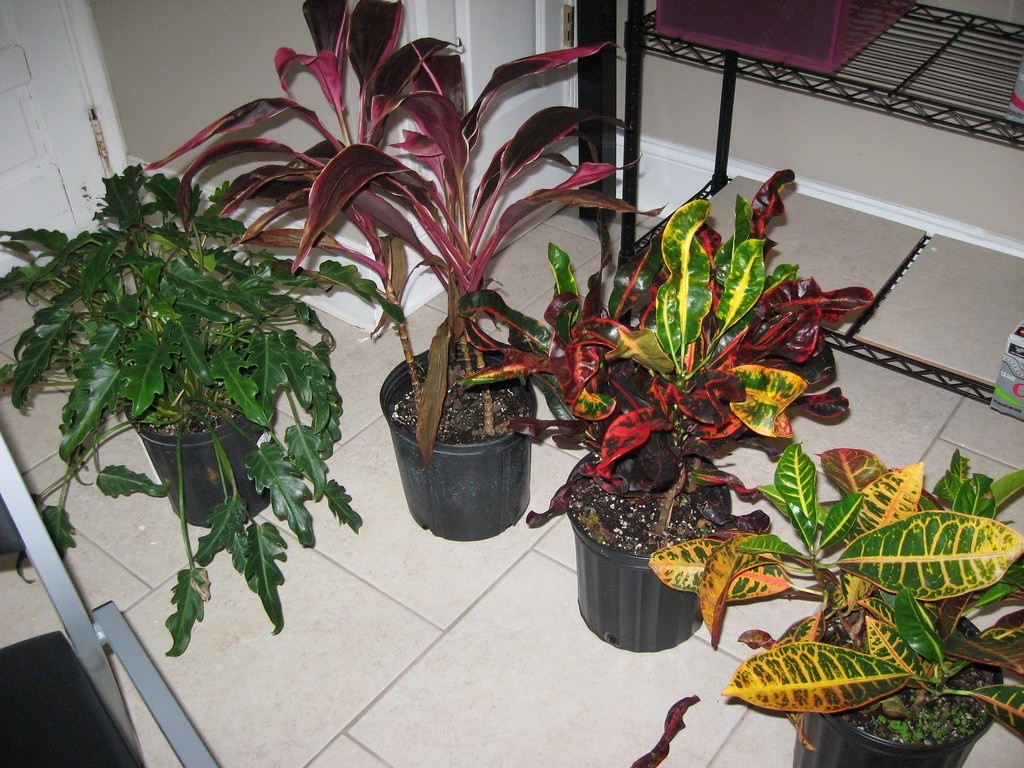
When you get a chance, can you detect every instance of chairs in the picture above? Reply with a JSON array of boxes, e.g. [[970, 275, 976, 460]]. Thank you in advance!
[[0, 421, 225, 768]]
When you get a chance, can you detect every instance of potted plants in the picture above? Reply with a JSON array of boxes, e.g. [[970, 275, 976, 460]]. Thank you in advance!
[[148, 0, 658, 541], [652, 443, 1024, 768], [0, 165, 412, 659], [464, 165, 873, 654]]
